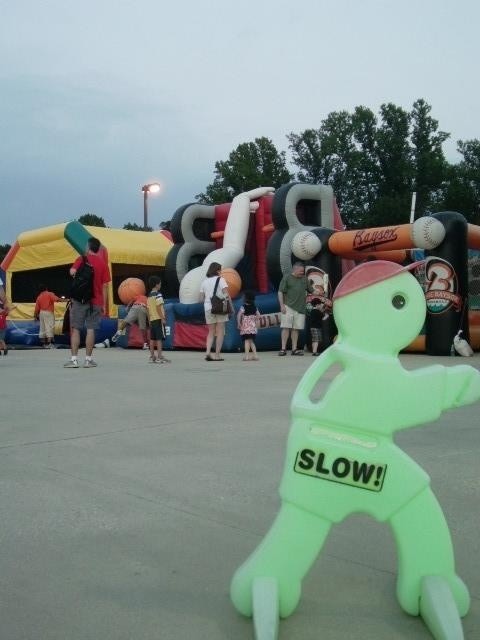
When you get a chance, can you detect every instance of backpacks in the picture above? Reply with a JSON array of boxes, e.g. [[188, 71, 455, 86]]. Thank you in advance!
[[70, 257, 94, 316]]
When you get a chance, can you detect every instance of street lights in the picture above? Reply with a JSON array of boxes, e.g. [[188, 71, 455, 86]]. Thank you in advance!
[[141, 182, 161, 228]]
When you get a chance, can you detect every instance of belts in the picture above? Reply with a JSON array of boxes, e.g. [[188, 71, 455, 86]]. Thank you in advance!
[[134, 304, 145, 307]]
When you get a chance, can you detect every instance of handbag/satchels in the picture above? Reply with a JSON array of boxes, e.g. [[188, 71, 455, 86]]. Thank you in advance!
[[211, 296, 231, 315]]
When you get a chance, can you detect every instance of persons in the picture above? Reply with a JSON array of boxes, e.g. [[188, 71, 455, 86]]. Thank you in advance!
[[278, 262, 316, 356], [199, 262, 234, 361], [0, 278, 9, 316], [34, 284, 63, 349], [310, 298, 325, 355], [0, 304, 17, 355], [64, 238, 112, 368], [112, 296, 149, 349], [147, 276, 171, 364], [237, 292, 260, 361]]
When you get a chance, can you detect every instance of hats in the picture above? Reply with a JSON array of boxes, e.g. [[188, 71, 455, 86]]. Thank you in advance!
[[311, 298, 324, 304]]
